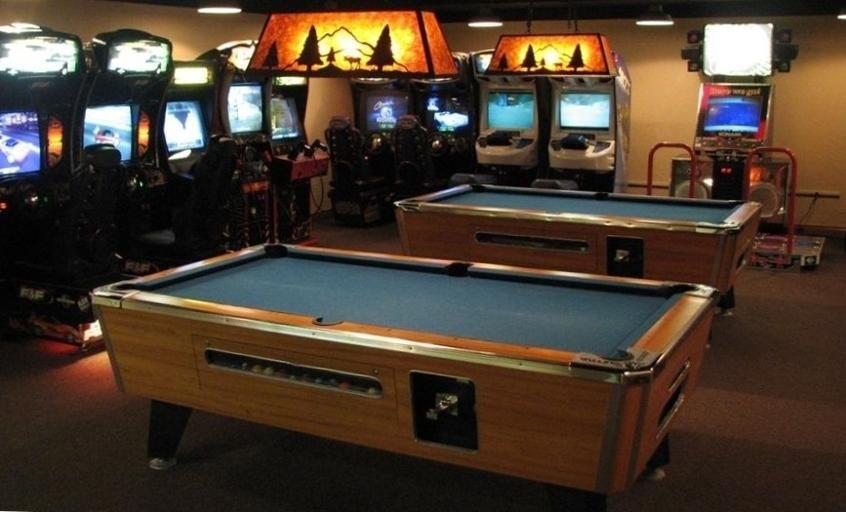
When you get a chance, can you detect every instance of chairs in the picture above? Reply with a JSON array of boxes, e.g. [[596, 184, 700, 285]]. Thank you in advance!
[[323, 114, 389, 226], [0, 141, 128, 313], [128, 132, 244, 262], [389, 113, 450, 220]]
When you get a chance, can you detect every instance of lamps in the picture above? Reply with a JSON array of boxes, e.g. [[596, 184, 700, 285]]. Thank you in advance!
[[467, 0, 504, 28], [635, 0, 675, 26], [197, 0, 243, 14], [241, 0, 459, 82], [484, 0, 619, 76]]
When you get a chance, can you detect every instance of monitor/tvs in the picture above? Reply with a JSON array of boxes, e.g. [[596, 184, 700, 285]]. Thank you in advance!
[[422, 91, 472, 133], [486, 90, 536, 132], [82, 103, 135, 164], [365, 94, 410, 132], [162, 98, 209, 153], [226, 84, 264, 134], [0, 109, 42, 179], [269, 96, 304, 141], [702, 93, 765, 134], [703, 21, 774, 78], [558, 91, 612, 132]]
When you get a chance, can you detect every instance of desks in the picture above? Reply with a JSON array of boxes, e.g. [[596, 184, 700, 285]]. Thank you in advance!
[[87, 238, 720, 512], [391, 181, 762, 345]]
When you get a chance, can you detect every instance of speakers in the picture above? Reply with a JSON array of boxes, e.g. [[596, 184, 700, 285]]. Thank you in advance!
[[774, 43, 800, 60], [747, 157, 790, 219], [687, 31, 702, 44], [774, 28, 794, 44], [775, 60, 792, 73], [681, 46, 702, 60], [670, 155, 715, 199], [686, 60, 701, 72]]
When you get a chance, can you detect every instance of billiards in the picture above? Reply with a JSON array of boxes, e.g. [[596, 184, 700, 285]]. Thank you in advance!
[[240, 361, 380, 395]]
